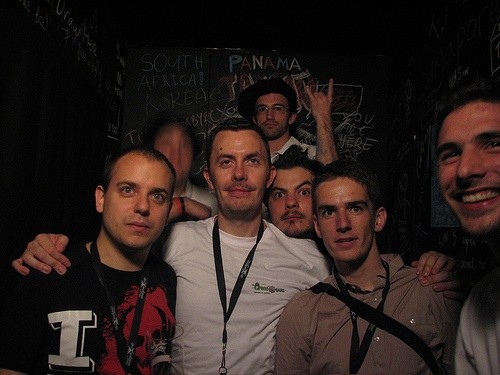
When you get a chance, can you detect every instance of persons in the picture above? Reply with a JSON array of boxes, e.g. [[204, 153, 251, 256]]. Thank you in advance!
[[143, 115, 218, 224], [275, 157, 465, 375], [0, 145, 177, 375], [262, 144, 457, 278], [11, 118, 463, 375], [432, 86, 500, 375], [252, 78, 339, 167]]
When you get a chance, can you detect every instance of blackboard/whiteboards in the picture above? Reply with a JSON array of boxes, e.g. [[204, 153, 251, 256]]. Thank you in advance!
[[121, 48, 391, 255]]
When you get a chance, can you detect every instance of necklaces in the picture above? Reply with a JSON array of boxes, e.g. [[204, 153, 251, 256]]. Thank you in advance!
[[85, 239, 153, 375], [328, 260, 394, 374], [207, 217, 265, 344]]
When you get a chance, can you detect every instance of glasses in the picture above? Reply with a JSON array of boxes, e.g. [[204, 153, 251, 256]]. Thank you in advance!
[[257, 104, 292, 115]]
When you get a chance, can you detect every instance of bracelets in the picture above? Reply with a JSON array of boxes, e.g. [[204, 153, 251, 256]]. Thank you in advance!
[[174, 196, 188, 216]]
